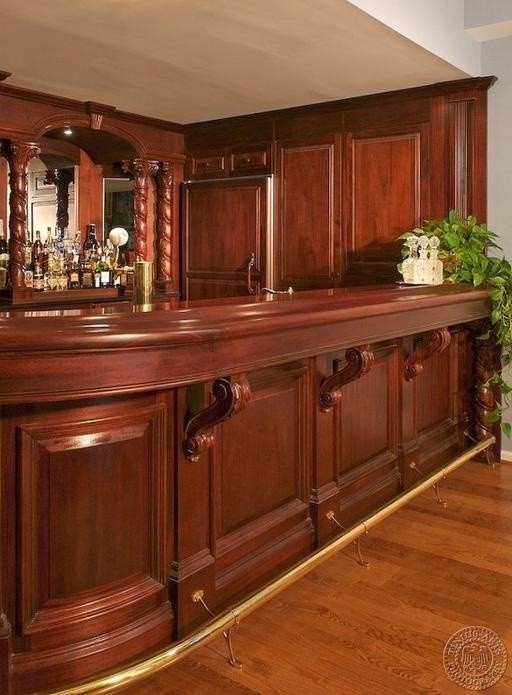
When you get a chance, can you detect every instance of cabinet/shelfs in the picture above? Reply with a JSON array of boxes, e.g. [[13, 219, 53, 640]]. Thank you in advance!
[[184, 76, 498, 300]]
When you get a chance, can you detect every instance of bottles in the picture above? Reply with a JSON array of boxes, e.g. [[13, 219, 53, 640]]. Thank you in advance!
[[0, 219, 127, 292], [402, 236, 443, 284]]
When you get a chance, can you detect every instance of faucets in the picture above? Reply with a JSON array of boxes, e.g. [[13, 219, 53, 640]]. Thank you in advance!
[[258, 286, 294, 295], [112, 262, 154, 314]]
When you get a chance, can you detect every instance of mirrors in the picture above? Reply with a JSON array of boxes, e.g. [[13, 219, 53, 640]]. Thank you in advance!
[[19, 114, 145, 290]]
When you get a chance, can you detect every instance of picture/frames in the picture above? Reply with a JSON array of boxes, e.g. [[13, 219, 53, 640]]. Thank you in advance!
[[101, 177, 136, 254]]
